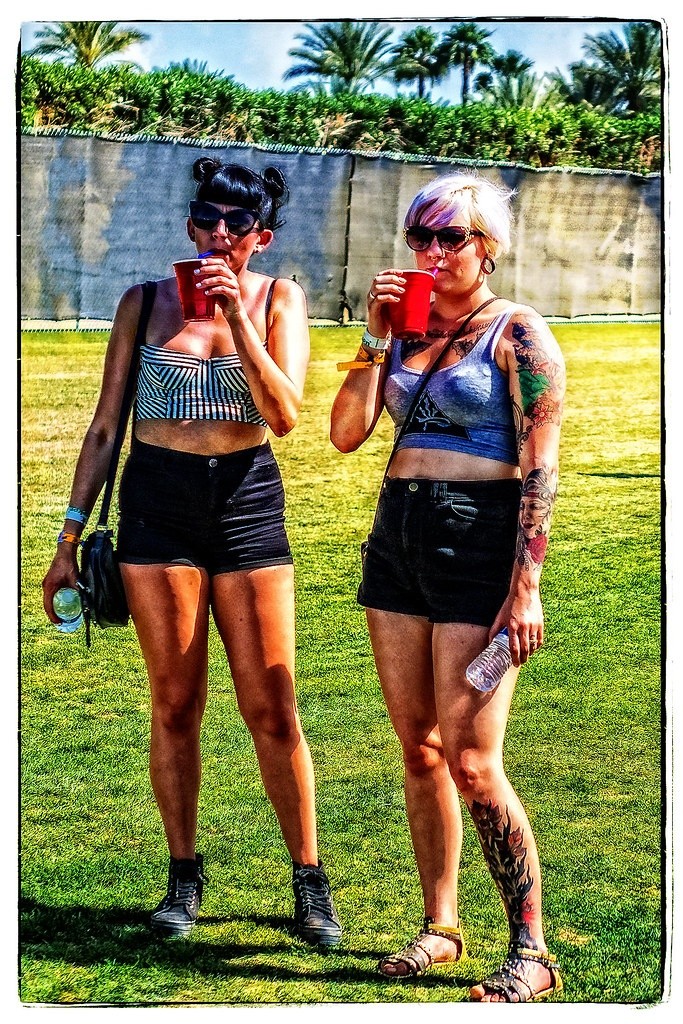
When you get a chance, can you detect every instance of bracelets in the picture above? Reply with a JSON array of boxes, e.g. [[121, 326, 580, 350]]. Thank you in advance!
[[336, 345, 385, 370], [66, 507, 89, 525], [362, 327, 392, 350], [57, 530, 81, 545]]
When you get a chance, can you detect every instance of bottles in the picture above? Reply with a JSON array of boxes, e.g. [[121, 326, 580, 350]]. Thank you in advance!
[[466, 626, 513, 693], [50, 586, 83, 634]]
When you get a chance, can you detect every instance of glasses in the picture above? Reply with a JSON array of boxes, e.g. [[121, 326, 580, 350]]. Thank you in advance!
[[188, 201, 260, 237], [402, 225, 484, 253]]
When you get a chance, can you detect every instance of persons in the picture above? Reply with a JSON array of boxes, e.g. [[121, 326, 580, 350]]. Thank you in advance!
[[328, 168, 565, 1002], [42, 157, 344, 951]]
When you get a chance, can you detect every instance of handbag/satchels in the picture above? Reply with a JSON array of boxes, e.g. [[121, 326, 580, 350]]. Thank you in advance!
[[76, 530, 129, 648]]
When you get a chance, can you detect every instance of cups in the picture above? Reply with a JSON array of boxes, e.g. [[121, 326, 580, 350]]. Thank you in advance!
[[171, 259, 216, 323], [387, 270, 436, 341]]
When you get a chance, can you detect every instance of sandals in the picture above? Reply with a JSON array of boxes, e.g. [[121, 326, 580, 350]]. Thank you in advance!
[[378, 915, 466, 978], [469, 941, 563, 1003]]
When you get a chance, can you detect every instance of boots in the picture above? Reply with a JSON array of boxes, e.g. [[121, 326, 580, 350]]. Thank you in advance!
[[292, 861, 342, 946], [150, 853, 209, 938]]
